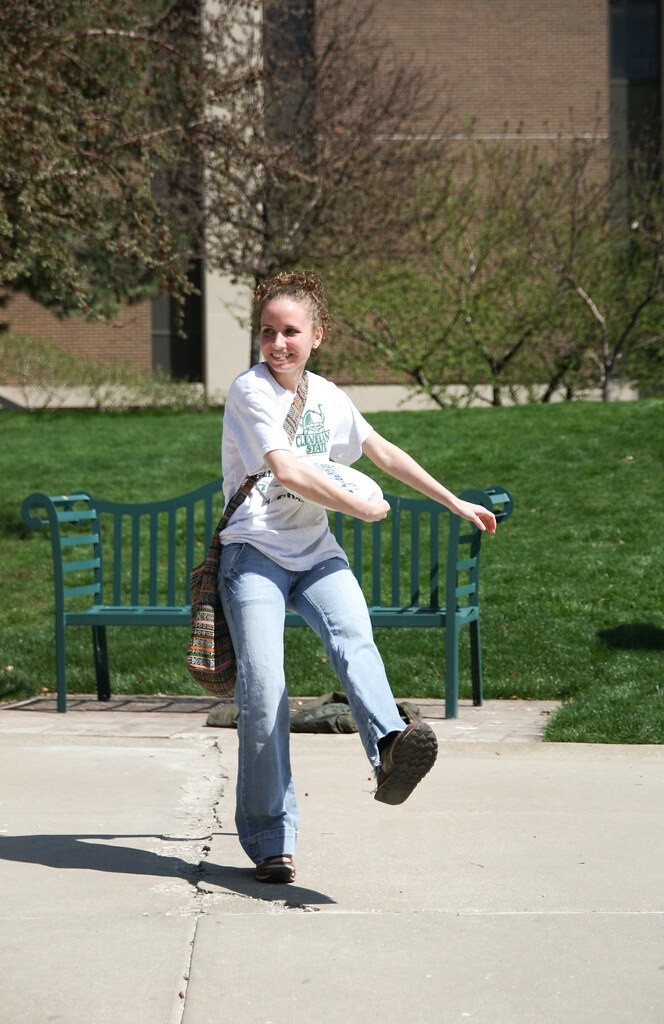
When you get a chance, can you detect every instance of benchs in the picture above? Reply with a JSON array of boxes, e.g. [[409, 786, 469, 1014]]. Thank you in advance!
[[20, 479, 511, 721]]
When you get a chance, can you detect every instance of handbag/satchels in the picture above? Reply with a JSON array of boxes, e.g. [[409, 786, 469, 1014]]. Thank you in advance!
[[186, 558, 235, 697]]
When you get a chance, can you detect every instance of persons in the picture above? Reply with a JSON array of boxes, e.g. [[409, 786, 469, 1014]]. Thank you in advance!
[[218, 269, 497, 887]]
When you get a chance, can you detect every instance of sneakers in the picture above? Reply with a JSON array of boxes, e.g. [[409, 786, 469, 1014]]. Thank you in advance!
[[372, 720, 438, 804], [255, 854, 295, 884]]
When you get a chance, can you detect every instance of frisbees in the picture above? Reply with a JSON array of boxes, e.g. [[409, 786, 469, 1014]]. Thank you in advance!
[[277, 457, 384, 516]]
